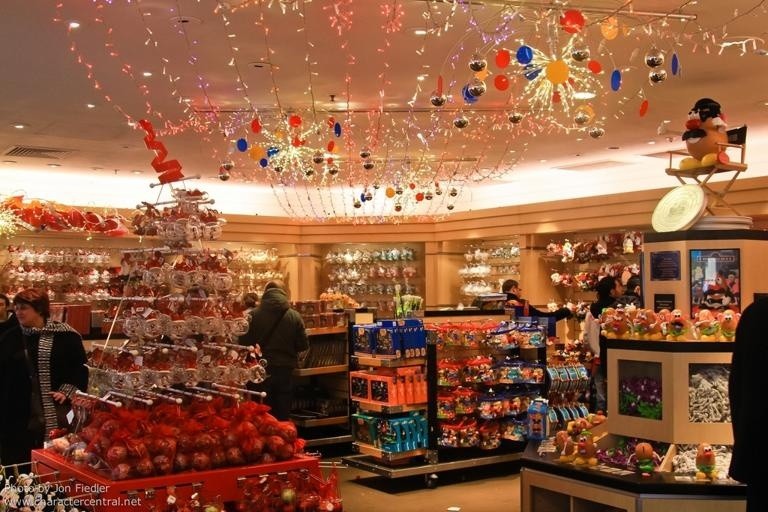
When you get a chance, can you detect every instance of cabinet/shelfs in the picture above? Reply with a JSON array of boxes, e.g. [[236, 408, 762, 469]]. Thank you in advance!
[[294, 328, 354, 427], [348, 353, 428, 462]]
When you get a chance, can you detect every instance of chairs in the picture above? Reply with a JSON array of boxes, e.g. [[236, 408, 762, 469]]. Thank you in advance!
[[664, 123, 751, 218]]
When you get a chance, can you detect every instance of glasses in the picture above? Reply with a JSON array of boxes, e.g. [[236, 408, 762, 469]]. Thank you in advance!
[[14, 305, 35, 311]]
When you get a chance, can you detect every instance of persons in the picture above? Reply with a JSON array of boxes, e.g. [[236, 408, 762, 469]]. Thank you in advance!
[[1, 294, 16, 336], [241, 292, 258, 310], [499, 279, 577, 323], [608, 276, 641, 311], [590, 275, 632, 317], [243, 280, 310, 417], [0, 287, 89, 484], [729, 284, 767, 512]]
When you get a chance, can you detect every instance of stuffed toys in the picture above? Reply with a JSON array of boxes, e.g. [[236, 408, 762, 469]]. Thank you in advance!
[[548, 234, 636, 313], [656, 96, 730, 170], [556, 300, 745, 488], [348, 316, 589, 464]]
